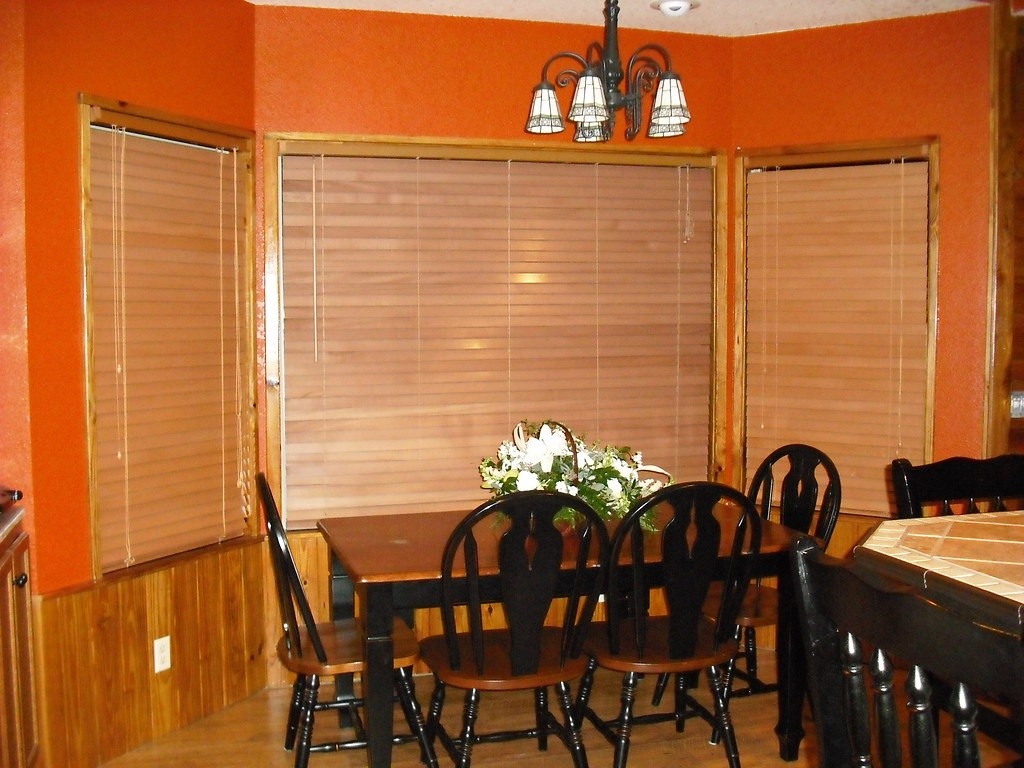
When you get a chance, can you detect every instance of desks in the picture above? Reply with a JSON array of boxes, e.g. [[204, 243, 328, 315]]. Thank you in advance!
[[317, 501, 823, 768], [829, 511, 1024, 713]]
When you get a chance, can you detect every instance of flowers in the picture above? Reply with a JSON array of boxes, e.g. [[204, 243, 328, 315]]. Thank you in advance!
[[478, 418, 673, 534]]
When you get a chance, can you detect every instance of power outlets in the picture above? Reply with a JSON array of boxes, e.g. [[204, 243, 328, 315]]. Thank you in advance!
[[155, 635, 172, 672]]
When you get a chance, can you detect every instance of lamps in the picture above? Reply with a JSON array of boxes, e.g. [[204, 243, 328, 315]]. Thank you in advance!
[[525, 0, 691, 142]]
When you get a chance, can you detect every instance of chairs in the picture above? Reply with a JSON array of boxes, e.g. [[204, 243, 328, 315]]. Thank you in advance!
[[254, 471, 441, 768], [702, 443, 841, 686], [892, 454, 1024, 522], [419, 492, 610, 768], [791, 535, 1024, 768], [569, 480, 763, 768]]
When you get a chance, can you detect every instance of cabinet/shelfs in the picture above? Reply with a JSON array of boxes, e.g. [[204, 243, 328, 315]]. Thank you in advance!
[[0, 505, 40, 768]]
